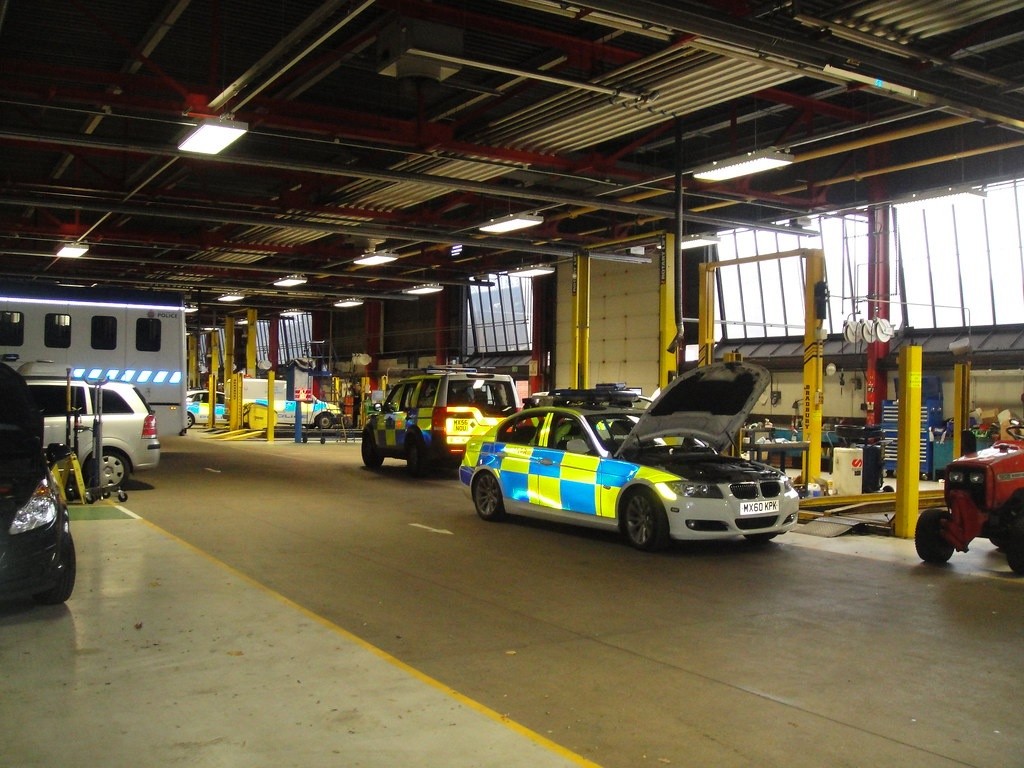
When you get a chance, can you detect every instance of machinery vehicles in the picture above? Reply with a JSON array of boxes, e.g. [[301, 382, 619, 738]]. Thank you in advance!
[[915, 424, 1024, 577]]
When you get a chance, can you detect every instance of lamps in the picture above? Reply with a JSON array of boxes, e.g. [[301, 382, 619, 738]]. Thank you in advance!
[[334, 297, 364, 308], [682, 234, 721, 250], [177, 111, 249, 155], [56, 243, 89, 258], [402, 284, 444, 294], [353, 248, 400, 266], [479, 198, 543, 233], [273, 273, 308, 287], [693, 98, 795, 181], [218, 292, 246, 302]]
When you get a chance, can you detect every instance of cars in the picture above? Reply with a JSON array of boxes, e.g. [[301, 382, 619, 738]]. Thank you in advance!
[[458, 358, 800, 550], [186, 388, 228, 427], [0, 361, 77, 617]]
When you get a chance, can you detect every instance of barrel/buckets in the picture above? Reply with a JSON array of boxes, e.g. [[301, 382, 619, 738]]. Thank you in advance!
[[807, 483, 821, 497]]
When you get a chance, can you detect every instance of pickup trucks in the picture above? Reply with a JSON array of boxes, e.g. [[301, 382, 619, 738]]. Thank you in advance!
[[225, 379, 343, 430], [361, 365, 522, 478], [14, 359, 162, 492]]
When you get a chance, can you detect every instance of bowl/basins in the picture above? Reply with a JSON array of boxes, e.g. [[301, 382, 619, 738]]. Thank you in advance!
[[834, 423, 866, 438]]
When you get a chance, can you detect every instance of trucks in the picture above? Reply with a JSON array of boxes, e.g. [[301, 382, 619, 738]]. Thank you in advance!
[[0, 296, 188, 439]]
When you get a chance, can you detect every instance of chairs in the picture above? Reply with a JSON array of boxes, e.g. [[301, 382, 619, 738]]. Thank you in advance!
[[604, 421, 635, 452]]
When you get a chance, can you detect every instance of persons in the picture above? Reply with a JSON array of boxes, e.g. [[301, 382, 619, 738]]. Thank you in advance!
[[352, 380, 362, 428]]
